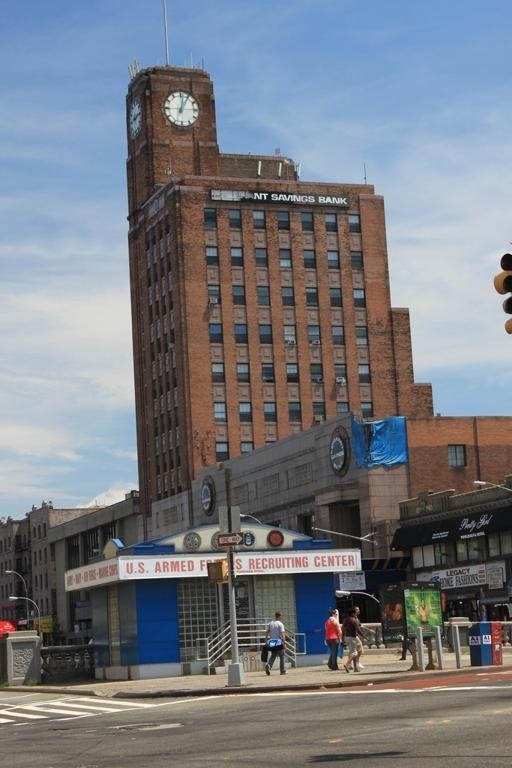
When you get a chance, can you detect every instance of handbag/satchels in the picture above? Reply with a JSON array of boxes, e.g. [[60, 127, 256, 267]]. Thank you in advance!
[[265, 638, 285, 650], [261, 649, 268, 662]]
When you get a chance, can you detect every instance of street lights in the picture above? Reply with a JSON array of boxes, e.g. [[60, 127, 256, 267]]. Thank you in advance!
[[5, 570, 41, 636]]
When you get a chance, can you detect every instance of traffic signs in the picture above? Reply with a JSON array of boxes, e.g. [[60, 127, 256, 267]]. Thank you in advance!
[[218, 533, 244, 546]]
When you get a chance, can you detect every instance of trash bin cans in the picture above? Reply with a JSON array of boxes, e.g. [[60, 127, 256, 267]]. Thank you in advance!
[[468, 622, 502, 666]]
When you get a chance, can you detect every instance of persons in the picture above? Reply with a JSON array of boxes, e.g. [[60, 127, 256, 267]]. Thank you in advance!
[[398, 615, 412, 661], [347, 606, 376, 669], [324, 609, 342, 670], [264, 610, 290, 675], [341, 608, 369, 673], [415, 598, 431, 626], [384, 599, 402, 621]]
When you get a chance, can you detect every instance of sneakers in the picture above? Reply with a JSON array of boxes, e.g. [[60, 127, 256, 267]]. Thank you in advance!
[[280, 671, 288, 675], [327, 663, 339, 671], [344, 664, 364, 672], [265, 665, 270, 675]]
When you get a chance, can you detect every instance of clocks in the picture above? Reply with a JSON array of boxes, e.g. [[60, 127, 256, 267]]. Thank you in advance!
[[129, 96, 142, 138], [161, 88, 201, 130]]
